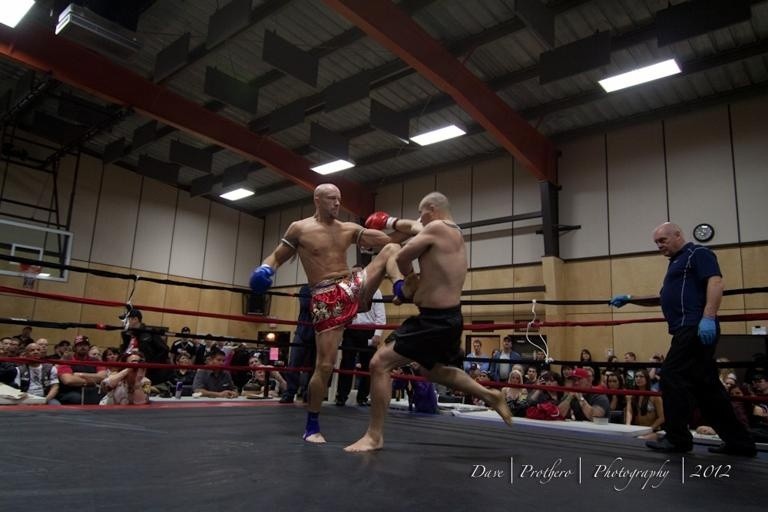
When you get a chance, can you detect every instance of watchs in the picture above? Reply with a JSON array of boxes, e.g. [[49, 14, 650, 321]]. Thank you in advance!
[[626, 294, 633, 300]]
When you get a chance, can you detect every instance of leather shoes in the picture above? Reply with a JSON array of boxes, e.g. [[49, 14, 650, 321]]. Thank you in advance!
[[645, 439, 695, 453], [709, 442, 759, 457]]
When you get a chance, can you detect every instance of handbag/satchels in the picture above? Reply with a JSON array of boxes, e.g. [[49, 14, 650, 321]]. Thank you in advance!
[[122, 328, 169, 363]]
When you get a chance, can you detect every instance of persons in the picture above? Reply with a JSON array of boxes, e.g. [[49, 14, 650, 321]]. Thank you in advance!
[[192, 349, 239, 399], [556, 369, 610, 421], [204, 334, 217, 360], [88, 346, 102, 360], [12, 326, 34, 346], [249, 183, 419, 444], [119, 308, 175, 384], [728, 384, 768, 430], [57, 335, 107, 405], [560, 362, 576, 383], [621, 352, 648, 392], [647, 352, 665, 393], [172, 353, 196, 386], [464, 340, 491, 374], [248, 356, 288, 396], [534, 350, 550, 371], [743, 353, 768, 385], [102, 346, 121, 377], [242, 365, 280, 394], [601, 369, 616, 388], [488, 350, 499, 369], [15, 349, 26, 366], [469, 364, 480, 402], [343, 191, 513, 452], [0, 337, 17, 367], [356, 335, 380, 407], [280, 284, 317, 404], [274, 348, 288, 366], [231, 342, 251, 394], [8, 338, 22, 357], [601, 356, 626, 389], [648, 407, 717, 441], [755, 375, 768, 395], [490, 337, 521, 383], [53, 340, 70, 360], [5, 343, 62, 406], [723, 373, 738, 393], [606, 374, 627, 423], [501, 370, 528, 418], [608, 222, 759, 459], [524, 367, 540, 391], [253, 346, 265, 362], [526, 372, 564, 406], [99, 350, 151, 406], [580, 349, 592, 363], [625, 369, 664, 440], [36, 339, 55, 366], [218, 336, 231, 355], [582, 367, 596, 386], [0, 342, 19, 389], [170, 327, 197, 365], [471, 367, 495, 407], [392, 360, 439, 414], [511, 364, 530, 385], [334, 264, 386, 407]]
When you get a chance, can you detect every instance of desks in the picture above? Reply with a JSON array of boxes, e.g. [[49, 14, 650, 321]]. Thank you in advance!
[[0, 382, 47, 405]]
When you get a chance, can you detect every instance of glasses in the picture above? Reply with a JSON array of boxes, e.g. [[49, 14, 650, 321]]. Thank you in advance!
[[479, 374, 487, 378], [725, 383, 734, 386], [730, 392, 743, 395], [131, 358, 146, 363], [753, 380, 767, 383], [539, 374, 548, 380], [608, 379, 619, 383], [636, 374, 645, 378]]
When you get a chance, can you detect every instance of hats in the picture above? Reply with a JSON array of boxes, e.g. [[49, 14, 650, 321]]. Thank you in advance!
[[480, 369, 489, 375], [503, 337, 512, 340], [725, 373, 737, 385], [752, 372, 767, 380], [567, 368, 592, 381], [182, 326, 190, 331], [653, 353, 664, 359], [75, 335, 90, 346], [602, 366, 618, 374], [119, 309, 141, 320], [469, 363, 481, 370]]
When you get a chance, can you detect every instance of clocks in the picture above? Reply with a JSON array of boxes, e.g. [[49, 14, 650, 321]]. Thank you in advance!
[[692, 223, 715, 242]]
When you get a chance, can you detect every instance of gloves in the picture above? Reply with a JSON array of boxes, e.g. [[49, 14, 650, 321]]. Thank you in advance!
[[249, 264, 274, 295], [365, 211, 398, 235], [698, 316, 717, 345], [608, 293, 631, 307]]
